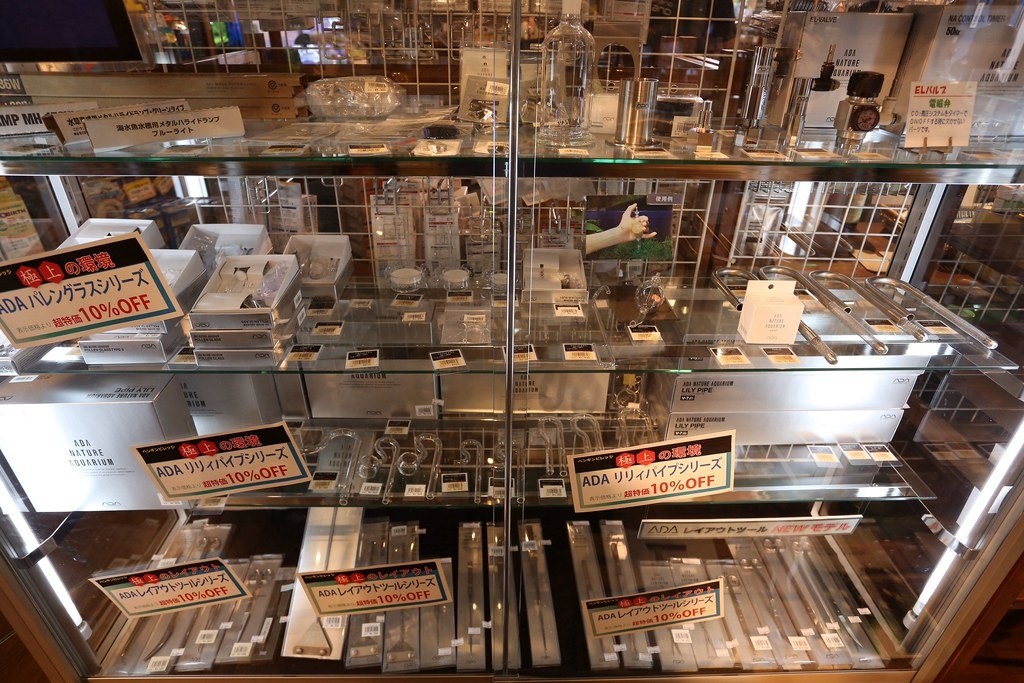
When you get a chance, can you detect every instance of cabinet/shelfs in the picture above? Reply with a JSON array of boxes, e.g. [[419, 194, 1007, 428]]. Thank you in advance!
[[0, 109, 1024, 683]]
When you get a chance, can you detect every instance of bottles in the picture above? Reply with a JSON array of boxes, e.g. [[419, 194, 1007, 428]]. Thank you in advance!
[[538, 0, 595, 144]]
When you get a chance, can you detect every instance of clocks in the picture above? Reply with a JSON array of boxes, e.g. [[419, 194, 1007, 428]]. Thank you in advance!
[[848, 102, 880, 133]]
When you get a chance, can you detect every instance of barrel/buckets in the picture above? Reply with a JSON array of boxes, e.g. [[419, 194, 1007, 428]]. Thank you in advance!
[[605, 78, 663, 150]]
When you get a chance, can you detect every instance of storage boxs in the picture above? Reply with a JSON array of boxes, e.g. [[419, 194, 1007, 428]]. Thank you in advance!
[[769, 3, 1024, 136], [642, 366, 918, 444], [0, 73, 307, 153], [0, 218, 610, 513]]
